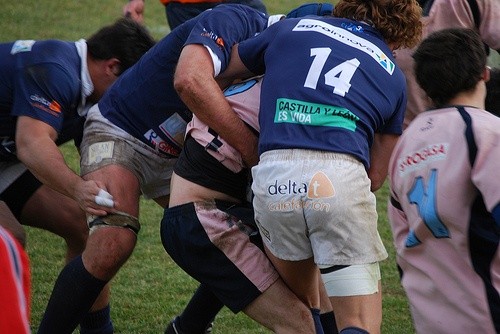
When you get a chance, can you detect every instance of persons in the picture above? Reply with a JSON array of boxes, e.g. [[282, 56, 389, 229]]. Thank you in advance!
[[387, 28, 500, 334], [123, 0, 269, 32], [37, 3, 335, 334], [0, 17, 158, 334], [160, 74, 316, 334], [1, 224, 32, 334], [213, 0, 424, 334], [393, 0, 500, 135]]
